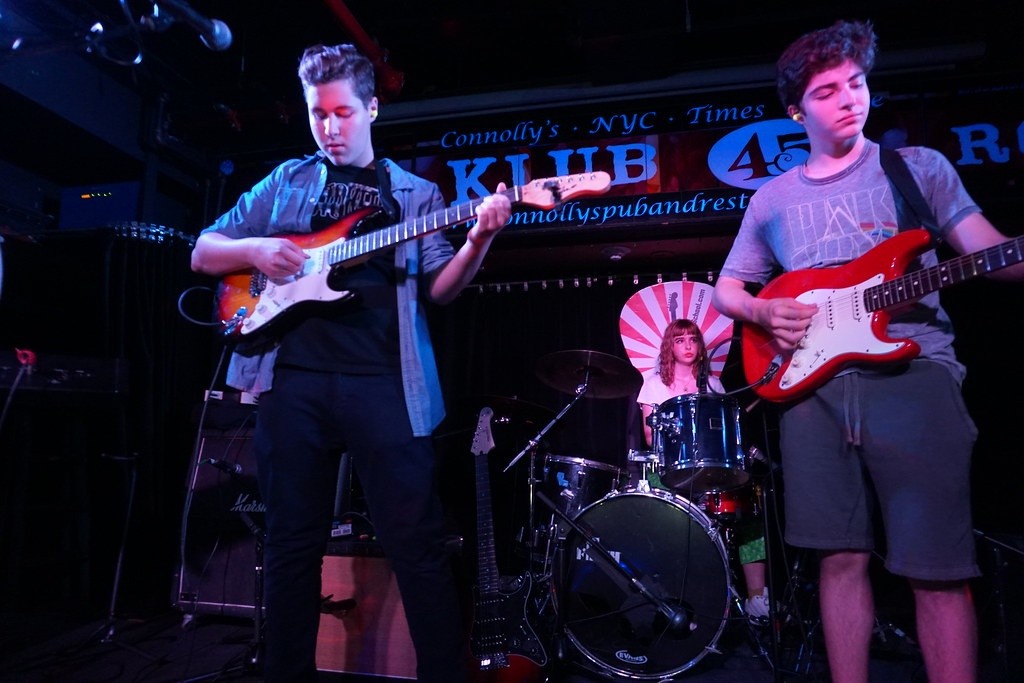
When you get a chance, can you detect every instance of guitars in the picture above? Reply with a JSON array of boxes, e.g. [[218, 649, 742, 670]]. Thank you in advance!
[[743, 228, 1024, 403], [214, 170, 611, 338], [464, 406, 547, 683]]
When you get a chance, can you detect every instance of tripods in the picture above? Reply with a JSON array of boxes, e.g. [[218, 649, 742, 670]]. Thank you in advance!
[[19, 451, 177, 673], [176, 476, 263, 683]]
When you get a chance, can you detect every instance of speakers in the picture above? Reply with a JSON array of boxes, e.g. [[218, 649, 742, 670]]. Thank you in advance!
[[313, 541, 420, 681], [173, 428, 264, 624]]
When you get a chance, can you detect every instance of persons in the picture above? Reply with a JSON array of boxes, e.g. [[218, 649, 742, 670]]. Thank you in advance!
[[637, 319, 726, 445], [712, 22, 1024, 683], [191, 45, 512, 683]]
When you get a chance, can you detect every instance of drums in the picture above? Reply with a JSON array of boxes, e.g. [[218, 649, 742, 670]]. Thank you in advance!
[[550, 486, 732, 679], [509, 454, 631, 563], [646, 394, 750, 492]]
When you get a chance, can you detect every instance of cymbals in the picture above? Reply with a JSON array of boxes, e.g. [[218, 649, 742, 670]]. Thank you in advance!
[[488, 392, 567, 424], [536, 350, 643, 398]]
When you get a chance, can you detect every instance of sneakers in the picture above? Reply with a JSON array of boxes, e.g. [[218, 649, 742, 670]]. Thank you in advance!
[[743, 586, 796, 630]]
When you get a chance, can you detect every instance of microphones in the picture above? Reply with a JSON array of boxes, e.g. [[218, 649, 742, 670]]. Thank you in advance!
[[661, 593, 685, 626], [209, 456, 242, 476]]
